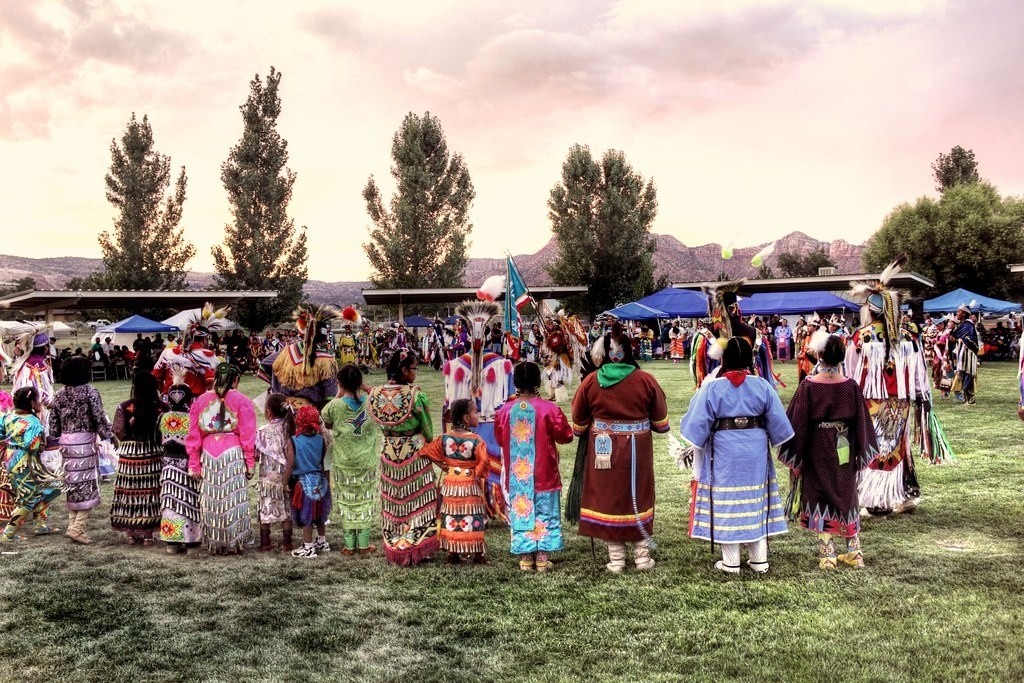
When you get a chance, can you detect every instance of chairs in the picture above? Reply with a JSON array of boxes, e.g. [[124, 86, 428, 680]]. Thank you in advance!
[[115, 359, 129, 380], [91, 361, 106, 382]]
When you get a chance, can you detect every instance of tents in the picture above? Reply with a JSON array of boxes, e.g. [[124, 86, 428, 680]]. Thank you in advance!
[[738, 291, 861, 319], [90, 315, 182, 352], [602, 287, 714, 320], [159, 307, 245, 333], [900, 287, 1023, 316]]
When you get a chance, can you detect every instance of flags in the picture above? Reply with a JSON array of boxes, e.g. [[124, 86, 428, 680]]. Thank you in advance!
[[504, 255, 537, 359]]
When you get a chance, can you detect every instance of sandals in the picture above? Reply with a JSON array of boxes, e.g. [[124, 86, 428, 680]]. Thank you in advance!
[[143, 536, 156, 548], [127, 537, 138, 547]]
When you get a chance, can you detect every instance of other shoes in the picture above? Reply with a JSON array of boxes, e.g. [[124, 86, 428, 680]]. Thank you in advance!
[[360, 543, 377, 555], [281, 545, 294, 553], [636, 559, 655, 571], [859, 507, 886, 520], [187, 547, 200, 556], [898, 497, 916, 513], [166, 544, 179, 554], [714, 561, 741, 574], [747, 559, 769, 574], [448, 554, 464, 564], [67, 530, 94, 544], [341, 547, 354, 555], [472, 556, 492, 565], [537, 559, 553, 572], [261, 541, 279, 551], [421, 556, 435, 564], [606, 562, 624, 575], [519, 558, 535, 570]]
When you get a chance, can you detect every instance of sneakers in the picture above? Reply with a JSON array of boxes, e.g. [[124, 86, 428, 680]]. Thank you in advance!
[[317, 542, 331, 552], [291, 542, 317, 559]]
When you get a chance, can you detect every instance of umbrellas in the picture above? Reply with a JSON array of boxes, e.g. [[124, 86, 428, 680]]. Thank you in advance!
[[391, 315, 434, 328]]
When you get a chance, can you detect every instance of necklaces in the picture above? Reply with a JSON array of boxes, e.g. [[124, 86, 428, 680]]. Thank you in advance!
[[817, 366, 838, 374]]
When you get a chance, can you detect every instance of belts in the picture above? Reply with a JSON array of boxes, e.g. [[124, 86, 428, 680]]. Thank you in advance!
[[713, 416, 766, 431]]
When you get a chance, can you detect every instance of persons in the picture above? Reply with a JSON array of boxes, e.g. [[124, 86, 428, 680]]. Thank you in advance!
[[249, 313, 1022, 368], [844, 262, 931, 518], [109, 333, 879, 576], [922, 299, 979, 408], [152, 302, 231, 404], [1017, 328, 1024, 420], [444, 298, 517, 528], [687, 277, 786, 541], [271, 300, 362, 528], [0, 319, 120, 544]]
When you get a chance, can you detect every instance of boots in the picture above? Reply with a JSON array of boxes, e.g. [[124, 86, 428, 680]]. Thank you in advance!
[[32, 503, 61, 534], [819, 533, 838, 573], [837, 535, 866, 569], [1, 507, 27, 547]]
